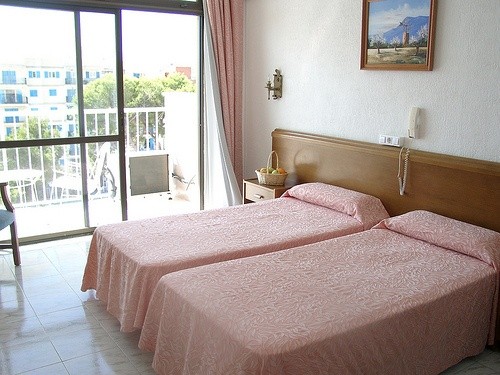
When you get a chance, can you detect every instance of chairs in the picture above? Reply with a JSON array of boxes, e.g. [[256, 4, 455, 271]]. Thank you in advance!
[[0, 182, 21, 266], [48, 142, 111, 212]]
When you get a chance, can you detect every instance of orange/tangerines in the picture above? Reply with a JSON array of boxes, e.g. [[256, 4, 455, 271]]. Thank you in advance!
[[276, 167, 286, 174]]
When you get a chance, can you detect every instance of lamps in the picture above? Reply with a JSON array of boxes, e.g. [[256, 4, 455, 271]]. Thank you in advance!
[[264, 68, 282, 100]]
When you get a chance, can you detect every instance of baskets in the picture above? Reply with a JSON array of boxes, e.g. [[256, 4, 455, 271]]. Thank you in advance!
[[255, 150, 288, 186]]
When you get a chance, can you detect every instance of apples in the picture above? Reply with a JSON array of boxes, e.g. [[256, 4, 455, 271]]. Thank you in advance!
[[260, 168, 267, 173], [268, 167, 278, 175]]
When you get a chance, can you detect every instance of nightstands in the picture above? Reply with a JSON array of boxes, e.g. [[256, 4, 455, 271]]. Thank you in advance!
[[242, 178, 304, 205]]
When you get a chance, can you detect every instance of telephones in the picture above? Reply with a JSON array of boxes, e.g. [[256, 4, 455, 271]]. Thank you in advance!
[[407, 107, 420, 139]]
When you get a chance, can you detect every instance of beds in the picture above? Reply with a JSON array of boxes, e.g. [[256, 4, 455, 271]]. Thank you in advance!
[[138, 209, 500, 375], [81, 182, 391, 333]]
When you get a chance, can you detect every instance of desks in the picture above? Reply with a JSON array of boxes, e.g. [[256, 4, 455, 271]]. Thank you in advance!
[[0, 169, 44, 218]]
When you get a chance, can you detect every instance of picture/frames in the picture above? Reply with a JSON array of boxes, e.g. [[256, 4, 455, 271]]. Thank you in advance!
[[360, 0, 437, 71]]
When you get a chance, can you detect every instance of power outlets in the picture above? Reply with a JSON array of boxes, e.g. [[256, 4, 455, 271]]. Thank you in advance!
[[379, 135, 405, 148]]
